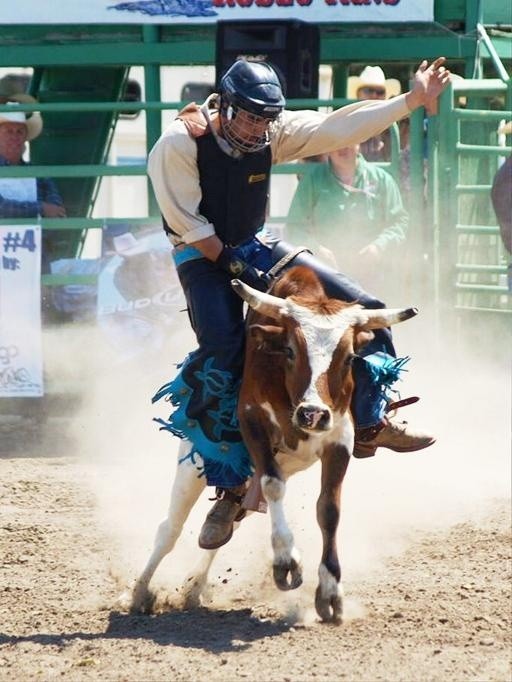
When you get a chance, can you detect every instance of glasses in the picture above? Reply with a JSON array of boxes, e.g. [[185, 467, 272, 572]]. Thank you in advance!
[[359, 86, 386, 96]]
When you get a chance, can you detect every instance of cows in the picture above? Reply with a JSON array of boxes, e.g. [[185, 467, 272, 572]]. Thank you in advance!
[[116, 265, 418, 625]]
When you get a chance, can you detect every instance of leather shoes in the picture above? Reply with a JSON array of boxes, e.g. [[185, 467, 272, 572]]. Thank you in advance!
[[199, 481, 248, 550], [352, 419, 435, 459]]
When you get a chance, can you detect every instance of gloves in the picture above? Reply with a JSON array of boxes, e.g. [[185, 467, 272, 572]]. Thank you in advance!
[[215, 245, 274, 292]]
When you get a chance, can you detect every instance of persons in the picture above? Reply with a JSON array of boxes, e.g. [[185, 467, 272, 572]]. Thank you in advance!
[[144, 56, 451, 550], [1, 102, 71, 322], [346, 65, 403, 161], [285, 143, 409, 295]]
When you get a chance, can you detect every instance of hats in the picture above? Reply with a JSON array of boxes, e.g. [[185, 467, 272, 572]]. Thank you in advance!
[[0, 101, 43, 142], [346, 66, 402, 100]]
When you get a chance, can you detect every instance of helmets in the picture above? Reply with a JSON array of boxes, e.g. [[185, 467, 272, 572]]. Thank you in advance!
[[219, 60, 287, 154]]
